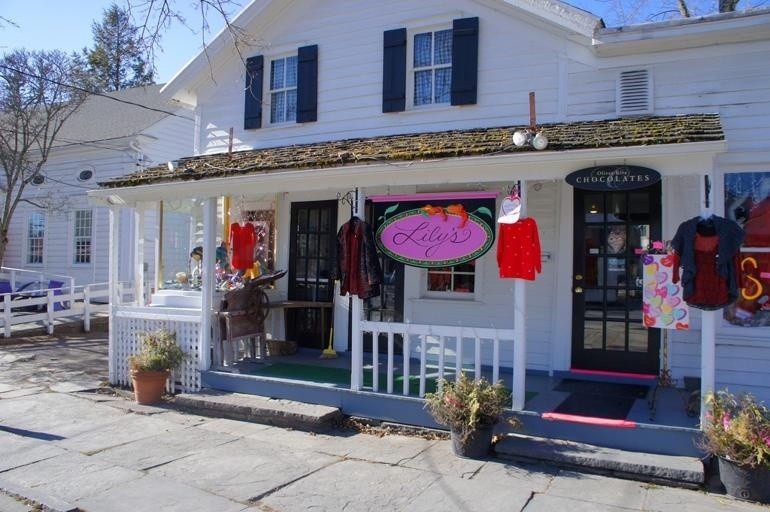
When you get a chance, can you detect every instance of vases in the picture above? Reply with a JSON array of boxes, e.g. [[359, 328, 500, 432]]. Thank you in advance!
[[717, 454, 770, 505]]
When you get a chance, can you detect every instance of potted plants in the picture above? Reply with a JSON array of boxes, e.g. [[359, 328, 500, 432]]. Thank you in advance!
[[126, 328, 192, 405]]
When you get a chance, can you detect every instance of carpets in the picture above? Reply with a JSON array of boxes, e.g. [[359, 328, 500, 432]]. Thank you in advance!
[[247, 363, 436, 393], [552, 379, 649, 399], [553, 390, 635, 419]]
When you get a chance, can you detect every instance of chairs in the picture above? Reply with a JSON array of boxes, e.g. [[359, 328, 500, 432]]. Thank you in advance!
[[0, 281, 65, 312]]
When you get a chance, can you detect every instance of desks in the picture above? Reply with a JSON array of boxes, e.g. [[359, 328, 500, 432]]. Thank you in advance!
[[150, 289, 249, 364]]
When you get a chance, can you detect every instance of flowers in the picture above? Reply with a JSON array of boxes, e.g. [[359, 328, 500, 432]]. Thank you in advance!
[[422, 371, 524, 446], [691, 384, 770, 471]]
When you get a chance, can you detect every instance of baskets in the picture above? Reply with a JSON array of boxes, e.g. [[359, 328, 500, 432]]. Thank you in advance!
[[266, 341, 297, 355]]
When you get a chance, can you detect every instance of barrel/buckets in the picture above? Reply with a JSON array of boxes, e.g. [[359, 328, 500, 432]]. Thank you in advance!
[[268, 340, 284, 355]]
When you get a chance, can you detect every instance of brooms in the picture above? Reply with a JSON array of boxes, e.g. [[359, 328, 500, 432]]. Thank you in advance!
[[320, 317, 339, 359]]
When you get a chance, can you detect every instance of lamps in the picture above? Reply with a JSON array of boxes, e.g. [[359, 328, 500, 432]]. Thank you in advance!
[[512, 126, 549, 150], [168, 161, 179, 173], [590, 204, 598, 214]]
